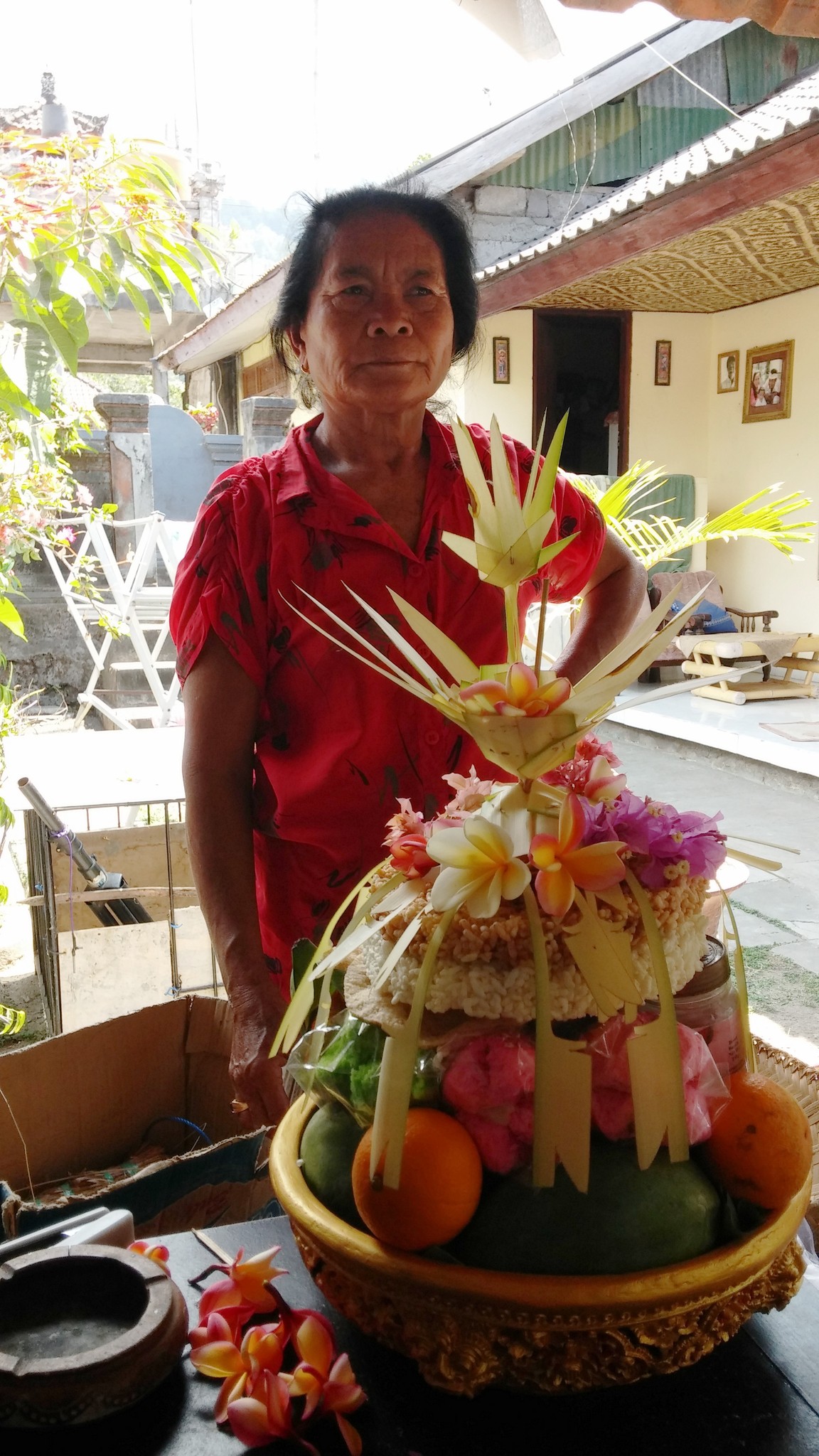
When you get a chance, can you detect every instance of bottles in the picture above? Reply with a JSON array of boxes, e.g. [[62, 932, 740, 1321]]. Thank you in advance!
[[665, 935, 751, 1086]]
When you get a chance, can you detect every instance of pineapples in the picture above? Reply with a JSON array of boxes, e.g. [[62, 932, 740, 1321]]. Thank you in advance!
[[352, 1107, 482, 1250]]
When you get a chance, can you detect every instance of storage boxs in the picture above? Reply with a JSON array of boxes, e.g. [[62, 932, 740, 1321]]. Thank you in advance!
[[0, 990, 305, 1243]]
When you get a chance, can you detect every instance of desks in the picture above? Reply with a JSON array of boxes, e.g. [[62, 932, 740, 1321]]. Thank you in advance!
[[0, 1210, 819, 1456], [675, 630, 819, 705]]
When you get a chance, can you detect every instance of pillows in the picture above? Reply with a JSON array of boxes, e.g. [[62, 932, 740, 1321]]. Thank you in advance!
[[671, 598, 738, 634]]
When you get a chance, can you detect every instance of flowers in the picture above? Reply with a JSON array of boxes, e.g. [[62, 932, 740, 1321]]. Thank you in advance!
[[119, 1228, 366, 1456], [383, 654, 725, 922], [183, 401, 219, 434]]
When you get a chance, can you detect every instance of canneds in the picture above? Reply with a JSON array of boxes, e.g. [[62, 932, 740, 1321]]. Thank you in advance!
[[638, 935, 746, 1077]]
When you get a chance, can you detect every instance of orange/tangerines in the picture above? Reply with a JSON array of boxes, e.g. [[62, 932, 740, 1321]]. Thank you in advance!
[[692, 1070, 813, 1210]]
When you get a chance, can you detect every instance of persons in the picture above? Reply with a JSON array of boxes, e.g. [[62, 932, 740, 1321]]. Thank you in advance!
[[750, 369, 782, 407], [721, 356, 736, 389], [170, 189, 647, 1133]]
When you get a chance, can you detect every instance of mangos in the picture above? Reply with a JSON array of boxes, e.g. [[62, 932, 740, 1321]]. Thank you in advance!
[[436, 1145, 723, 1273], [299, 1103, 361, 1222]]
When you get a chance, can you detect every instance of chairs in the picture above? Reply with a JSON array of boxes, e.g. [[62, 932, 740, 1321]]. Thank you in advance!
[[37, 509, 196, 729], [638, 569, 778, 682]]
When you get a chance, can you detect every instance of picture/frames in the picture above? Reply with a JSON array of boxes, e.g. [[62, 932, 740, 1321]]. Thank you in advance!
[[654, 340, 671, 386], [741, 338, 795, 425], [717, 350, 739, 394], [492, 336, 511, 384]]
